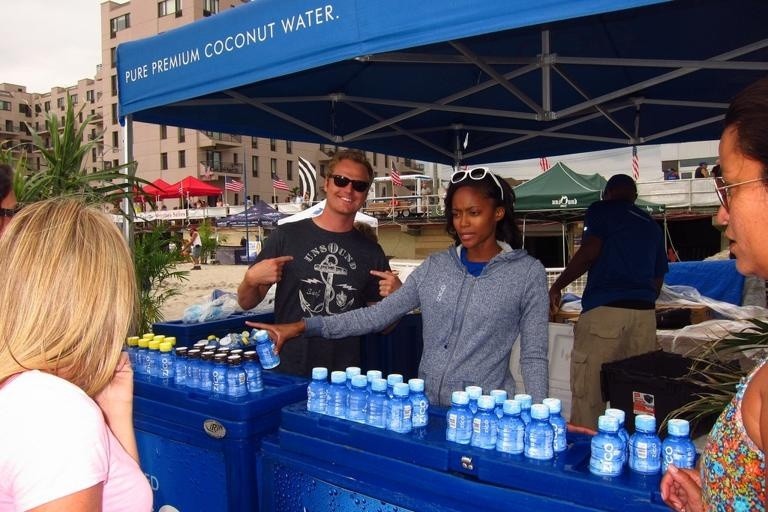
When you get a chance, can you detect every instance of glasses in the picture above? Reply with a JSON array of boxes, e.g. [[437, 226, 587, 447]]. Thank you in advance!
[[713, 178, 766, 213], [449, 167, 504, 202], [0, 202, 25, 217], [328, 173, 371, 192]]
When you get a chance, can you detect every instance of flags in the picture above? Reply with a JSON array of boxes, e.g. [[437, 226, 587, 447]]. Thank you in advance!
[[272, 172, 289, 191], [633, 146, 640, 181], [225, 176, 244, 193], [540, 158, 550, 172], [391, 161, 402, 187]]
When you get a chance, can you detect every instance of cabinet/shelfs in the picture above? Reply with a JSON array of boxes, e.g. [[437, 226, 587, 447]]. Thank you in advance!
[[252, 397, 701, 512], [133, 372, 304, 512], [151, 313, 271, 348]]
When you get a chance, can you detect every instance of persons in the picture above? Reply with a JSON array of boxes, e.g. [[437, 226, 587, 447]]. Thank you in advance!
[[290, 189, 304, 203], [183, 228, 204, 270], [245, 166, 550, 406], [238, 149, 403, 373], [660, 79, 767, 511], [667, 168, 680, 180], [421, 184, 431, 218], [0, 197, 154, 512], [712, 158, 721, 177], [695, 162, 709, 178], [548, 174, 669, 431], [194, 199, 205, 208], [215, 198, 223, 206], [169, 240, 176, 268], [386, 194, 400, 218], [1, 163, 18, 243], [246, 196, 253, 205]]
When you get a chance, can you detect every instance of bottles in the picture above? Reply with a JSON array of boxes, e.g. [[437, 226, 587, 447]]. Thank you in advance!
[[628, 413, 662, 475], [125, 330, 175, 379], [306, 366, 431, 434], [604, 407, 630, 466], [252, 329, 281, 370], [588, 414, 626, 479], [662, 418, 697, 477], [171, 328, 264, 399], [445, 385, 568, 460]]
